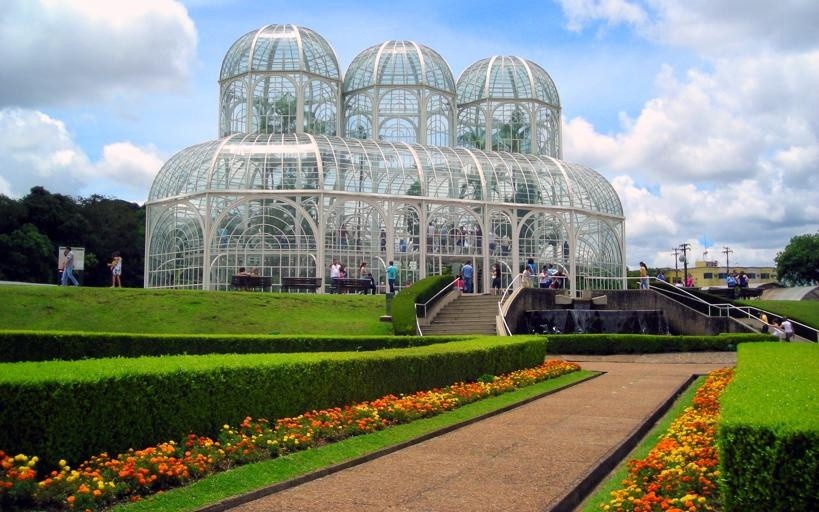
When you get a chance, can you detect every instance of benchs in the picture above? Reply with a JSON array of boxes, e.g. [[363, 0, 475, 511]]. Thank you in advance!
[[281, 277, 322, 293], [686, 287, 702, 290], [738, 287, 763, 301], [231, 275, 273, 293], [708, 287, 736, 299], [329, 278, 372, 295]]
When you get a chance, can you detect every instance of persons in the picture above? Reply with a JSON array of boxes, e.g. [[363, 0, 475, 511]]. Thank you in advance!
[[639, 261, 695, 290], [217, 223, 229, 250], [454, 260, 473, 293], [726, 269, 750, 300], [522, 258, 568, 288], [491, 263, 503, 289], [107, 250, 124, 288], [358, 262, 376, 295], [238, 267, 260, 292], [760, 311, 793, 343], [327, 258, 347, 294], [385, 261, 397, 294], [60, 246, 80, 286]]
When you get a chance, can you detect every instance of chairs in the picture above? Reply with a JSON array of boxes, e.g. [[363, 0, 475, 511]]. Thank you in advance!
[[554, 294, 575, 309], [591, 294, 608, 310]]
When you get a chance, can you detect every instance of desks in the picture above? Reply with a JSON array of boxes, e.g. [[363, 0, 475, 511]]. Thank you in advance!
[[573, 297, 592, 304]]
